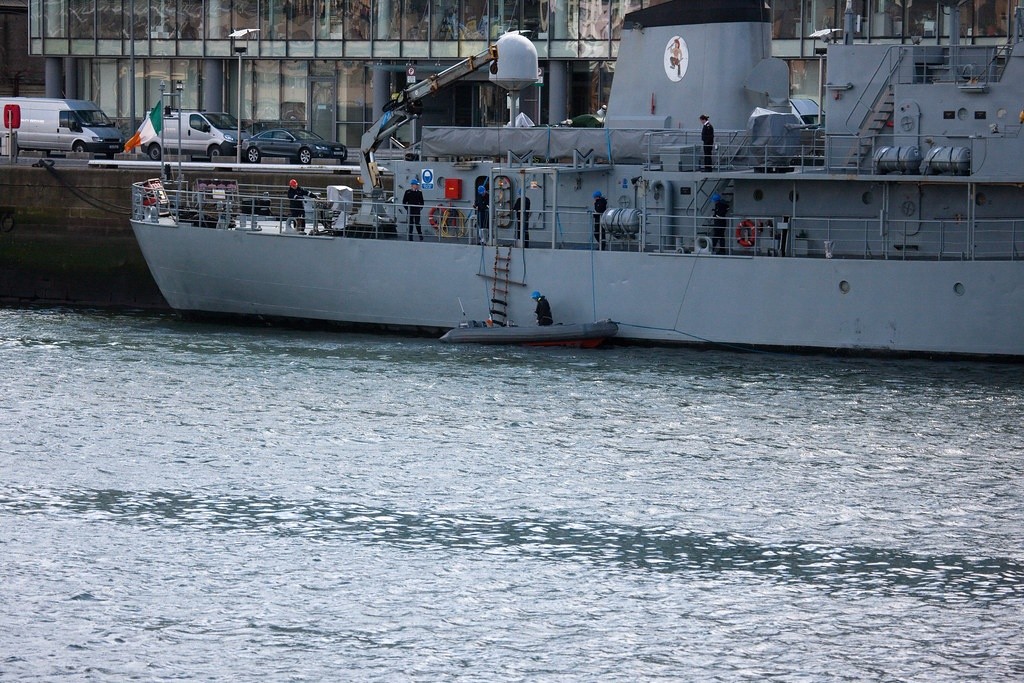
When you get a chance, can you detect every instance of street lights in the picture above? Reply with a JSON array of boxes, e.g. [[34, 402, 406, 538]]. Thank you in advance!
[[229, 27, 261, 163]]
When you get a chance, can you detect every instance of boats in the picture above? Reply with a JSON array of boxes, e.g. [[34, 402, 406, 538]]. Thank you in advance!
[[440, 317, 618, 343], [131, 0, 1024, 361]]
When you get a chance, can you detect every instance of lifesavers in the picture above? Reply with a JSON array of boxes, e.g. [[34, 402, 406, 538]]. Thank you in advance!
[[428, 205, 449, 230], [736, 220, 755, 247]]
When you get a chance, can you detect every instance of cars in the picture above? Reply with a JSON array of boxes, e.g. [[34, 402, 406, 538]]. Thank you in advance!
[[241, 127, 347, 165]]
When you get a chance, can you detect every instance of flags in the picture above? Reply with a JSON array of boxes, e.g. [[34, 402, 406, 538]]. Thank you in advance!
[[124, 100, 164, 154]]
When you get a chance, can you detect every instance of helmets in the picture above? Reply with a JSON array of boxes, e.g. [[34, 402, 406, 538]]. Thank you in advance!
[[518, 188, 526, 193], [593, 191, 602, 198], [531, 291, 540, 299], [713, 194, 721, 202], [290, 179, 298, 186], [411, 179, 420, 185], [478, 186, 485, 194]]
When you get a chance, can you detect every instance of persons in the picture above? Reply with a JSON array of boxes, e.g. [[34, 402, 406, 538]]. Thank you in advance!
[[711, 193, 729, 255], [288, 179, 319, 231], [402, 179, 424, 241], [700, 115, 714, 172], [531, 291, 553, 326], [592, 191, 607, 250], [473, 185, 489, 246], [514, 188, 530, 248]]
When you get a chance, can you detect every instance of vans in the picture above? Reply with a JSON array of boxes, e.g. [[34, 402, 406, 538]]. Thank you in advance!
[[141, 109, 249, 161], [0, 97, 125, 157]]
